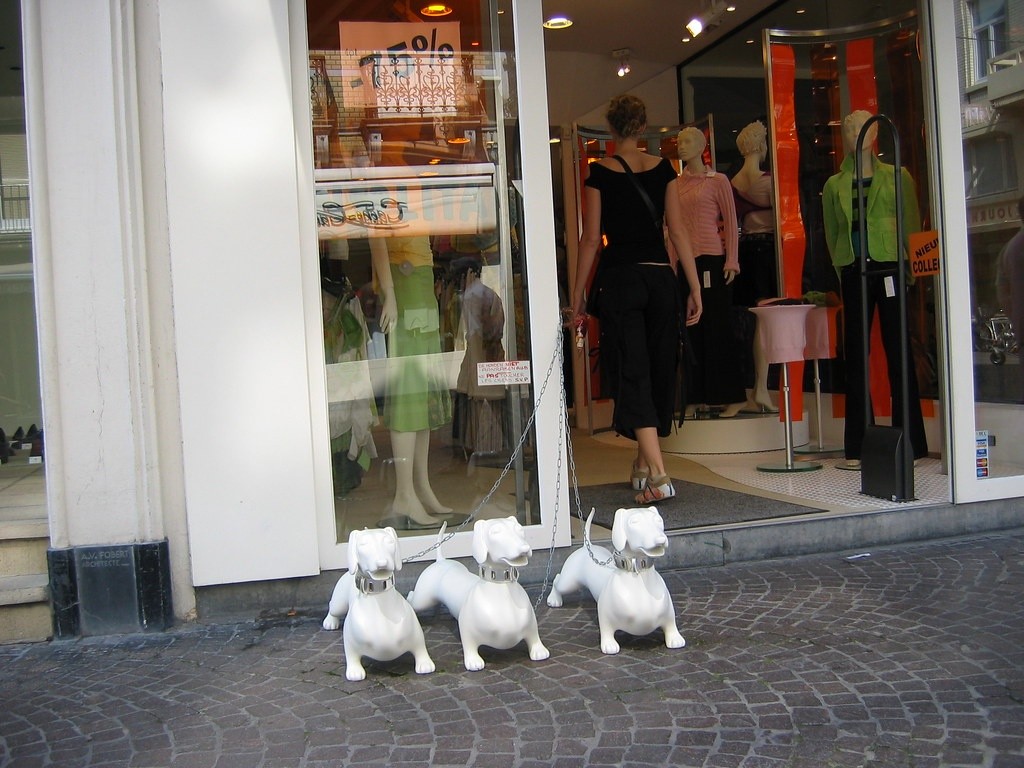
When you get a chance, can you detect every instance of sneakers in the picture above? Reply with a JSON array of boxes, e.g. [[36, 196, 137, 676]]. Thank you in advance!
[[632, 475, 678, 504], [630, 459, 652, 491]]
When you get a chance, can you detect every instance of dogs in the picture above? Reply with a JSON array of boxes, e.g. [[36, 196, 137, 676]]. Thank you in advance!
[[406, 515, 551, 672], [546, 507, 687, 656], [322, 526, 437, 681]]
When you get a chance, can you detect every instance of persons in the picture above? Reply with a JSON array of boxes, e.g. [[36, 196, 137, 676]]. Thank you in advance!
[[365, 97, 454, 527], [446, 256, 525, 468], [572, 94, 703, 503], [666, 126, 739, 413], [821, 110, 928, 472], [729, 121, 781, 415]]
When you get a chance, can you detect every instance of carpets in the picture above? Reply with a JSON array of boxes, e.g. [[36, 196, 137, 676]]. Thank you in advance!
[[477, 456, 532, 472], [509, 478, 830, 531]]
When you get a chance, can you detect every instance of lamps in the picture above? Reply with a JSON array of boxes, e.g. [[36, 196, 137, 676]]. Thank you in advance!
[[685, 0, 730, 37]]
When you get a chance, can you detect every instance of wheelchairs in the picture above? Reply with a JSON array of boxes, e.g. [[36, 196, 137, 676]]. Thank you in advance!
[[972, 311, 1018, 365]]
